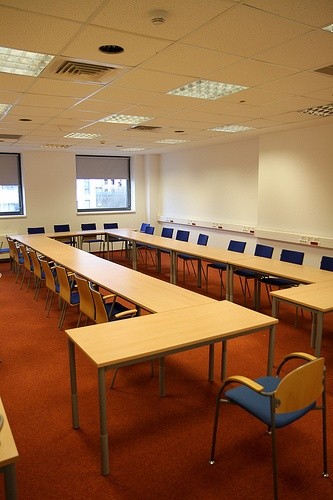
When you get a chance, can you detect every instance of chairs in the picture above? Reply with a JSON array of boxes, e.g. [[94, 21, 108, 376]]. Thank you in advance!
[[0, 222, 333, 500]]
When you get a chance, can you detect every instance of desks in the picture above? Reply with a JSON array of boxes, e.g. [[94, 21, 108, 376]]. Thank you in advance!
[[106, 228, 333, 349], [42, 228, 126, 262], [65, 300, 279, 476], [270, 278, 333, 367], [9, 234, 222, 397]]
